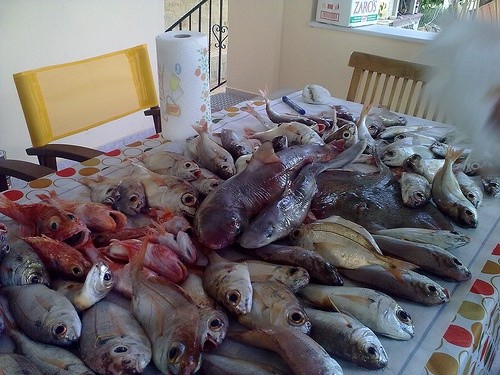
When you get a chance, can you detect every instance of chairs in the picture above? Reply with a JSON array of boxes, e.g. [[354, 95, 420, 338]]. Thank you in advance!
[[346, 51, 455, 125], [0, 158, 55, 191], [13, 43, 160, 171]]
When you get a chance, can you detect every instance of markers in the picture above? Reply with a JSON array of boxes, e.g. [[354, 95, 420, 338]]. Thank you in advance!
[[281, 96, 305, 115]]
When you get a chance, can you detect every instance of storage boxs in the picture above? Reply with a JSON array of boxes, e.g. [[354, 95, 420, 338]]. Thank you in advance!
[[316, 1, 378, 27]]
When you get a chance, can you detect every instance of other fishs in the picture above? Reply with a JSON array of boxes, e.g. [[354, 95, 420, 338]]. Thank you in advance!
[[191, 121, 236, 180], [83, 206, 200, 284], [256, 82, 326, 133], [0, 222, 50, 288], [296, 283, 416, 341], [225, 327, 345, 375], [128, 158, 200, 218], [374, 227, 471, 252], [232, 243, 344, 286], [212, 128, 247, 163], [78, 175, 147, 216], [243, 121, 327, 147], [318, 100, 437, 149], [298, 296, 389, 371], [201, 339, 295, 374], [240, 103, 280, 130], [110, 230, 230, 375], [431, 145, 479, 230], [190, 165, 225, 196], [0, 326, 98, 375], [333, 264, 451, 306], [288, 215, 421, 290], [46, 259, 115, 312], [8, 284, 82, 345], [463, 150, 500, 199], [285, 113, 330, 128], [136, 149, 202, 181], [0, 192, 91, 250], [36, 190, 127, 232], [201, 243, 254, 315], [371, 234, 473, 283], [430, 142, 474, 164], [16, 233, 95, 282], [79, 300, 153, 375], [310, 144, 455, 232], [449, 161, 483, 209]]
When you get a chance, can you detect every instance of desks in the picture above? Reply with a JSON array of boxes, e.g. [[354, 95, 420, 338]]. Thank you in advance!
[[0, 90, 500, 375]]
